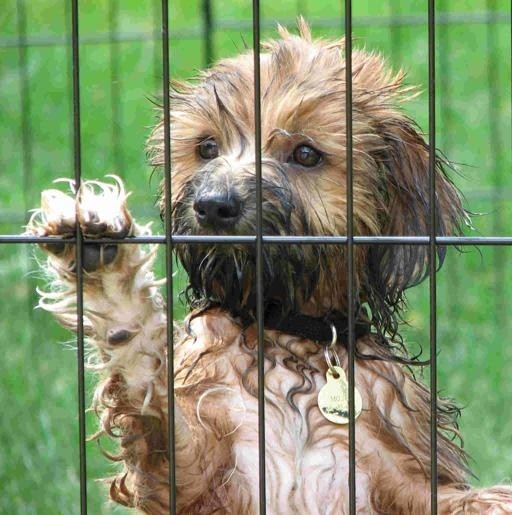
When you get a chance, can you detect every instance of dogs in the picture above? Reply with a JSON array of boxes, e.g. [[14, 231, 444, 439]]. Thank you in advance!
[[19, 14, 512, 515]]
